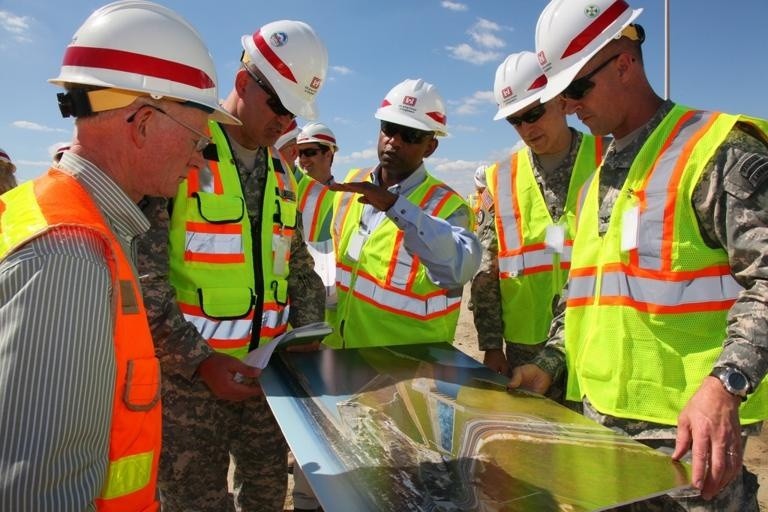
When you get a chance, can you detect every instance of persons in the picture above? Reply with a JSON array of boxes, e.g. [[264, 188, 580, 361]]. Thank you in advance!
[[295, 122, 339, 242], [470, 51, 614, 403], [504, 0, 768, 511], [272, 118, 302, 185], [330, 79, 484, 353], [0, 0, 243, 511], [131, 20, 325, 511]]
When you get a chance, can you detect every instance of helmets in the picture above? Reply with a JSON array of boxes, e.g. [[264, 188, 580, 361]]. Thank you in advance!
[[48, 0, 243, 126], [375, 79, 449, 136], [241, 19, 327, 120], [275, 122, 340, 152], [493, 51, 547, 121], [535, 0, 644, 104]]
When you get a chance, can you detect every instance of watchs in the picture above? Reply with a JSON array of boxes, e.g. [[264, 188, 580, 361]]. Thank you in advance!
[[712, 367, 753, 400]]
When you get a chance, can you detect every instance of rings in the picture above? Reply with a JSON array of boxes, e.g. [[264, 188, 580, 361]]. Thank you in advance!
[[729, 453, 743, 457]]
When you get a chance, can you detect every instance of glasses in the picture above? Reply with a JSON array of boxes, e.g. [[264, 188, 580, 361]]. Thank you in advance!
[[298, 148, 324, 157], [560, 55, 635, 100], [243, 62, 290, 116], [380, 121, 435, 143], [506, 103, 547, 124], [128, 105, 212, 152]]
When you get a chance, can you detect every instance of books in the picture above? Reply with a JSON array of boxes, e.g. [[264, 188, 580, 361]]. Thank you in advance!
[[229, 316, 333, 381]]
